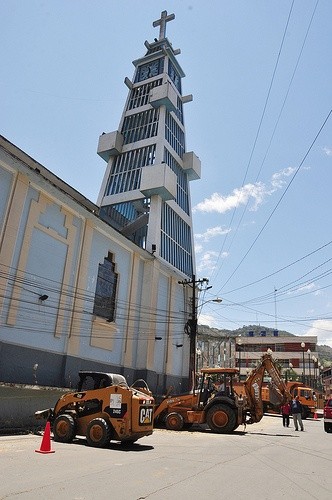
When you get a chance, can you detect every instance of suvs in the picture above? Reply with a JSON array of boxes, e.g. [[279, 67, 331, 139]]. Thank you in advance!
[[323, 397, 332, 433]]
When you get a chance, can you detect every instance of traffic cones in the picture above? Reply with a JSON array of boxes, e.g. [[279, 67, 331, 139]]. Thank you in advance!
[[35, 421, 56, 454], [312, 413, 320, 421]]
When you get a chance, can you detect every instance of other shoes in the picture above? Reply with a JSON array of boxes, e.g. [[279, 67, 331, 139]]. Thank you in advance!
[[301, 429, 303, 431], [286, 424, 288, 427], [295, 428, 298, 431]]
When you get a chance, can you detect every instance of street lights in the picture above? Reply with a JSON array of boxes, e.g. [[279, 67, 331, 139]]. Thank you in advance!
[[312, 354, 324, 392], [307, 349, 311, 387], [288, 362, 293, 379], [189, 298, 222, 395], [300, 341, 305, 388]]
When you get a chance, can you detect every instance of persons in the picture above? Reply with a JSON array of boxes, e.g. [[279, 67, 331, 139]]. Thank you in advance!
[[290, 396, 303, 431], [217, 380, 224, 392], [282, 400, 291, 427]]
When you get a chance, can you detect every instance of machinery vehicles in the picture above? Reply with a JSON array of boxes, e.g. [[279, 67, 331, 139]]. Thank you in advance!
[[34, 369, 156, 449], [151, 348, 317, 434]]
